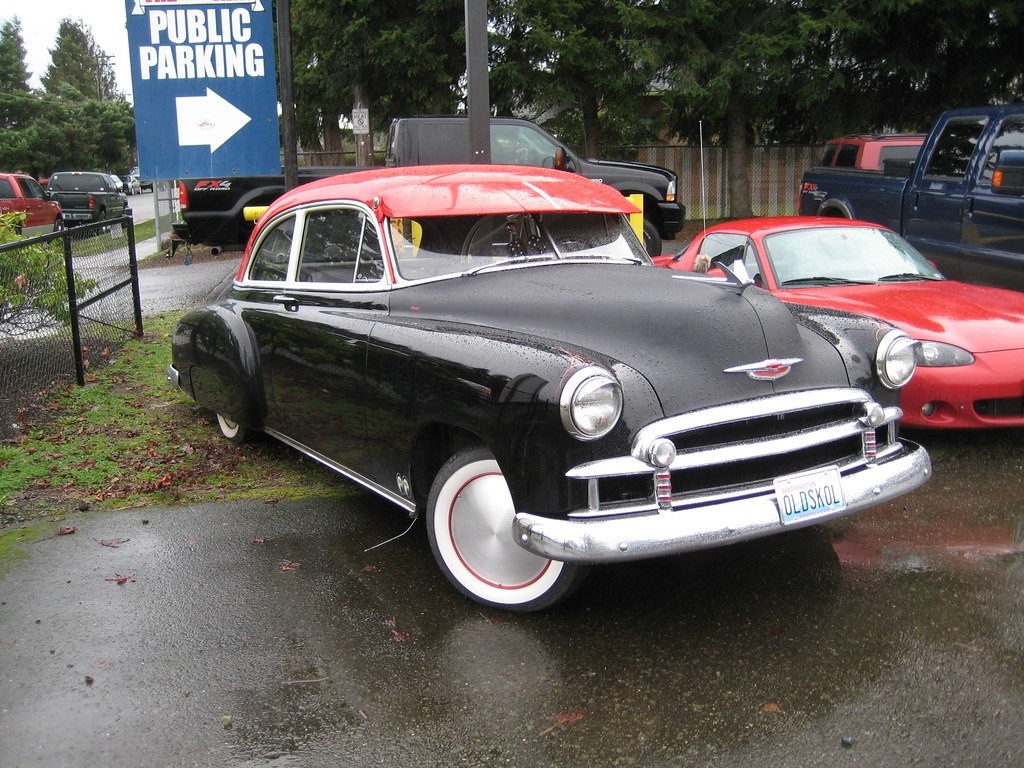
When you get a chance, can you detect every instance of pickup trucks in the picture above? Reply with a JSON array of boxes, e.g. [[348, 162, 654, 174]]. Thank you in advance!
[[160, 112, 687, 256], [797, 106, 1023, 292]]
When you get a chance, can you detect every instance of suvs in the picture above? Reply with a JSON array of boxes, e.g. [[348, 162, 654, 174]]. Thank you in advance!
[[129, 167, 153, 192], [817, 134, 929, 175], [0, 172, 65, 241], [46, 171, 130, 236]]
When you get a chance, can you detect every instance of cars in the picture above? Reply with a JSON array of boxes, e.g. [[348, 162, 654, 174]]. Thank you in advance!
[[119, 174, 142, 196], [646, 213, 1023, 439], [166, 164, 935, 613]]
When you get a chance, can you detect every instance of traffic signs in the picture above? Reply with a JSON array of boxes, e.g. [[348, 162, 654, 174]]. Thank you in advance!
[[122, 0, 283, 183]]
